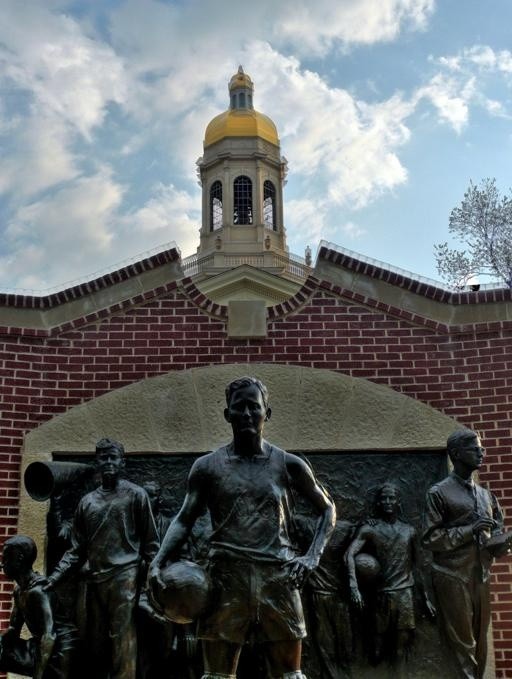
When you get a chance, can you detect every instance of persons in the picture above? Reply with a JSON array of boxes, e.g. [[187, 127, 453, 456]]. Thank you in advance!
[[291, 484, 436, 679], [0, 436, 170, 679], [147, 377, 337, 679], [421, 429, 512, 679]]
